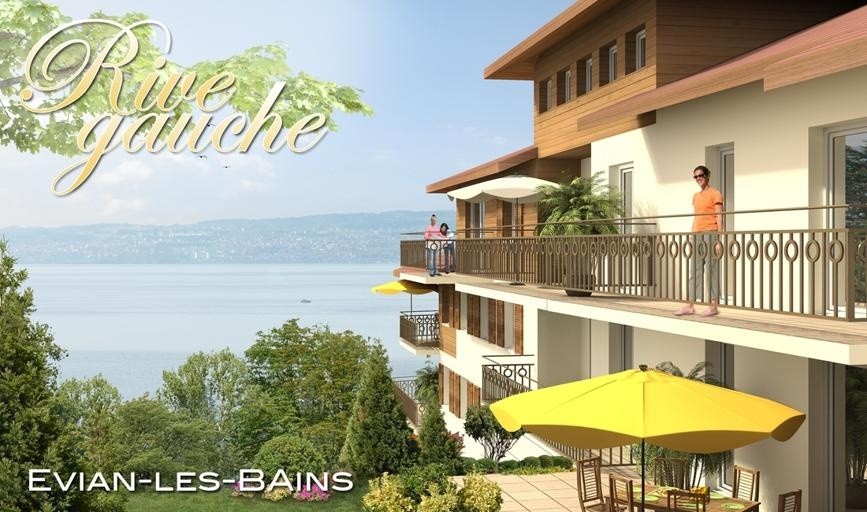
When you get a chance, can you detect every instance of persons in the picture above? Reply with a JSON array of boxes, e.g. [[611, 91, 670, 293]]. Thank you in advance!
[[425, 214, 447, 277], [675, 166, 724, 316], [440, 223, 456, 274]]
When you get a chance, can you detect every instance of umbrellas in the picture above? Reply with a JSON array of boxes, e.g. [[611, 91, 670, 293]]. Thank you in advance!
[[489, 365, 805, 512], [447, 171, 569, 281], [371, 280, 434, 315]]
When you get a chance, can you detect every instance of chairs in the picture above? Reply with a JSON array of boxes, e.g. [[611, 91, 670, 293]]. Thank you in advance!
[[577, 456, 802, 512]]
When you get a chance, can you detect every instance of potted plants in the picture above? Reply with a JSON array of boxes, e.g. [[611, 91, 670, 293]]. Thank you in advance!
[[846, 369, 867, 509], [532, 165, 629, 296]]
